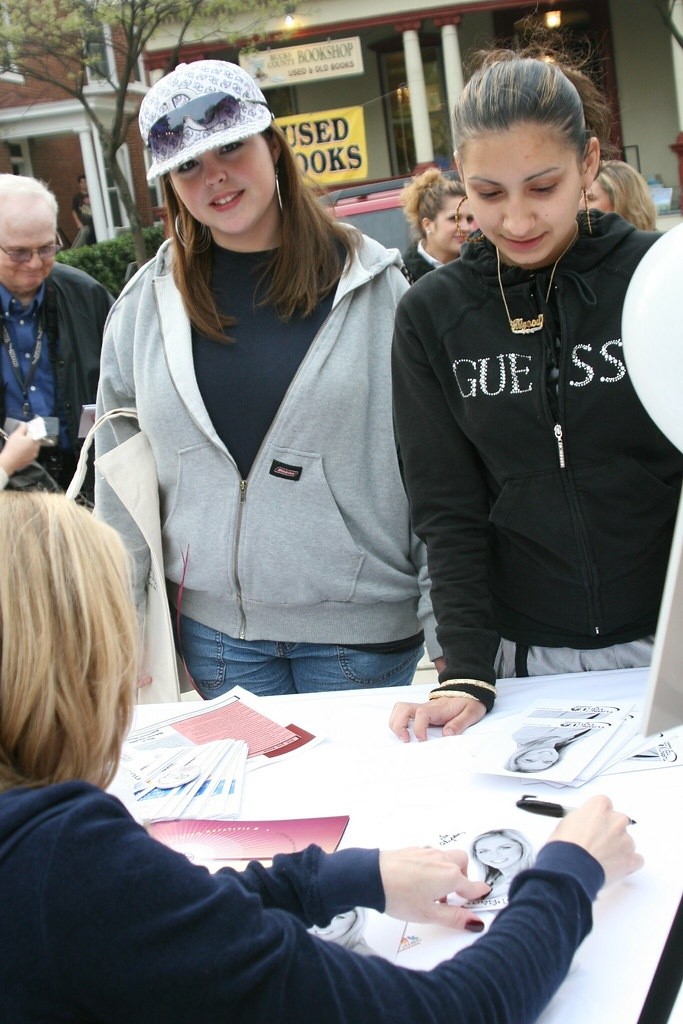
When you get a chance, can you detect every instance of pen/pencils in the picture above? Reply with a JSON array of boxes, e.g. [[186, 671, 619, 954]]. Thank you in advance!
[[517, 793, 638, 828]]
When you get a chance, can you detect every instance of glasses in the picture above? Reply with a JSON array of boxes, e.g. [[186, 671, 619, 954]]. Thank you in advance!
[[0, 232, 63, 262], [145, 91, 270, 159]]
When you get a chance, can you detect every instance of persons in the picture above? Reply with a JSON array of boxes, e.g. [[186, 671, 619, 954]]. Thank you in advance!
[[527, 710, 608, 720], [72, 176, 96, 245], [0, 173, 116, 514], [506, 726, 592, 773], [389, 56, 683, 743], [400, 167, 475, 281], [578, 161, 656, 232], [0, 491, 643, 1024], [0, 422, 41, 492], [472, 829, 537, 901], [90, 60, 445, 700], [306, 906, 375, 954]]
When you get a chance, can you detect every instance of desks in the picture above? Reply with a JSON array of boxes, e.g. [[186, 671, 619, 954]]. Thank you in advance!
[[107, 665, 683, 1024]]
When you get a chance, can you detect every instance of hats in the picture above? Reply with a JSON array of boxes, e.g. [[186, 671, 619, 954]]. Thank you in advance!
[[138, 59, 274, 181]]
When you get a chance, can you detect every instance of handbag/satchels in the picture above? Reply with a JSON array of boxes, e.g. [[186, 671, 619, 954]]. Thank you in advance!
[[65, 407, 181, 702]]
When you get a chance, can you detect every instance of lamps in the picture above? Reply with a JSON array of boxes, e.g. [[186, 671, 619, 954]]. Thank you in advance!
[[546, 10, 562, 29]]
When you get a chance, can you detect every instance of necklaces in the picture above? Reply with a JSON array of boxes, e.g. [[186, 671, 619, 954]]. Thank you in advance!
[[496, 220, 579, 334]]
